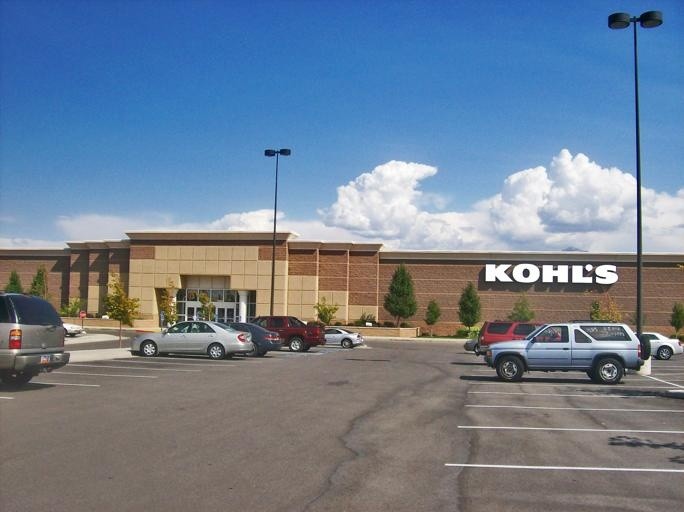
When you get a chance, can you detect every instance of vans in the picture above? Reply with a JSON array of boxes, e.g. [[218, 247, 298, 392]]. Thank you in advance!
[[0, 292, 70, 384]]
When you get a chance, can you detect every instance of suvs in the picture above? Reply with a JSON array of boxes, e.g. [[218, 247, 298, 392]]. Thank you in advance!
[[464, 319, 684, 384]]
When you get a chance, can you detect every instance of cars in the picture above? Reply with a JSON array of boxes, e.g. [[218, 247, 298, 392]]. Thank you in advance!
[[63, 322, 83, 336], [131, 316, 364, 360]]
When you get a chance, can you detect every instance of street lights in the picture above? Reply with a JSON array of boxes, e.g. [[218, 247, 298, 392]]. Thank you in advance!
[[608, 11, 664, 340], [263, 149, 291, 316]]
